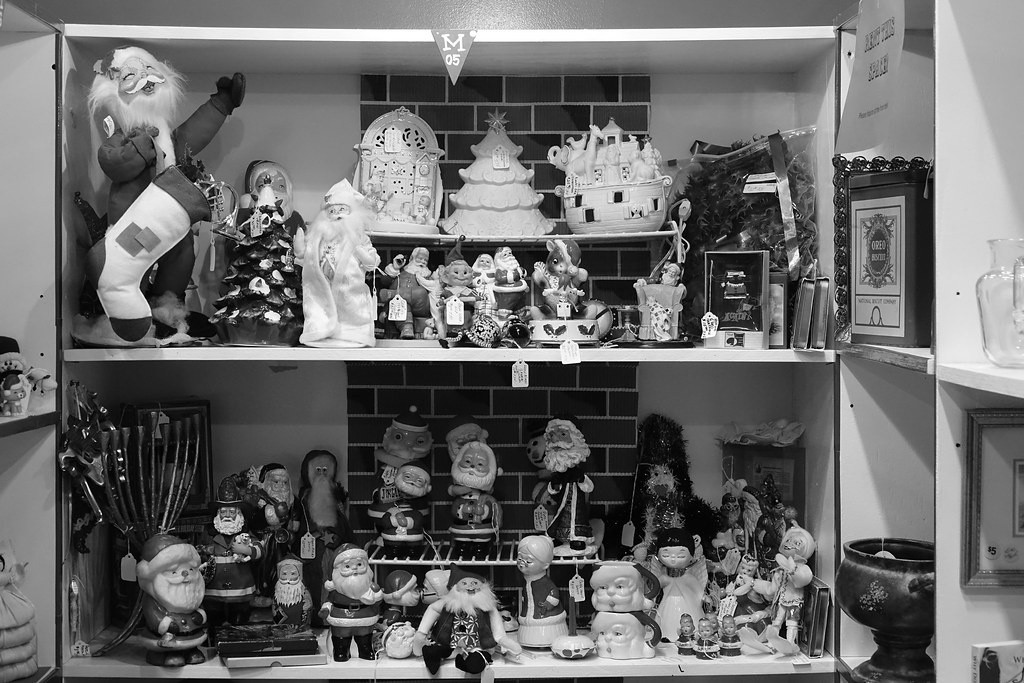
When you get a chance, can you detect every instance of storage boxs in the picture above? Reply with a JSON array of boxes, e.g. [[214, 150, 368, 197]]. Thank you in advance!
[[850, 170, 930, 348]]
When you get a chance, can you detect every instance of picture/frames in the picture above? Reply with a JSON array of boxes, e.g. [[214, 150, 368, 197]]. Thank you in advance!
[[960, 407, 1024, 594]]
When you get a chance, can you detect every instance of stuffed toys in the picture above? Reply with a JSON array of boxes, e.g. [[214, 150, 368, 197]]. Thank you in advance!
[[81, 42, 248, 347], [0, 336, 34, 421]]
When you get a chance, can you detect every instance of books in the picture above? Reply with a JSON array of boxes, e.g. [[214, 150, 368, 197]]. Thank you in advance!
[[790, 276, 831, 352]]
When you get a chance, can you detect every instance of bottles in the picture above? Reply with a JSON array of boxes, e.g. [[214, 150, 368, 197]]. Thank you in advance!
[[975, 238, 1024, 368]]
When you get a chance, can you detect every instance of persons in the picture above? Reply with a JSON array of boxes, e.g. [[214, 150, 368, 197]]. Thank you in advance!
[[119, 405, 817, 675], [207, 158, 531, 348], [659, 263, 681, 287]]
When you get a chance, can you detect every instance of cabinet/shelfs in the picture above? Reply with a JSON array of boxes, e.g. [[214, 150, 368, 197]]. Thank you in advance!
[[824, 0, 1023, 682], [59, 23, 846, 683], [0, 2, 64, 683]]
